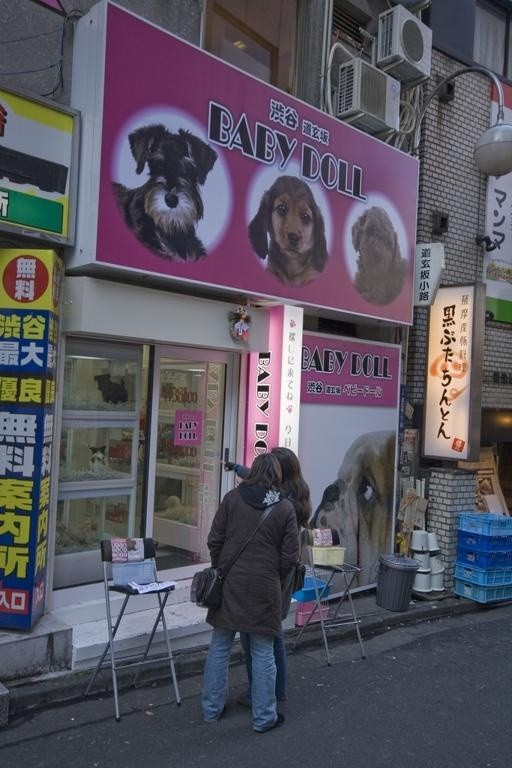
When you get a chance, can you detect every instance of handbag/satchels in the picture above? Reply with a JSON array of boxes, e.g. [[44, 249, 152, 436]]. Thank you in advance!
[[291, 563, 306, 594], [189, 565, 222, 611]]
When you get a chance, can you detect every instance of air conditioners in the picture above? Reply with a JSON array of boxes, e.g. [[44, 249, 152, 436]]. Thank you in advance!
[[377, 5, 432, 83], [337, 58, 400, 138]]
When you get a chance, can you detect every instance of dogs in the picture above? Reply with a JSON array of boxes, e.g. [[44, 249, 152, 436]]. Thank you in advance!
[[55, 517, 98, 549], [88, 445, 108, 476], [94, 373, 128, 405], [351, 206, 408, 307], [247, 174, 329, 287], [297, 430, 396, 597], [110, 121, 220, 264], [154, 495, 199, 525]]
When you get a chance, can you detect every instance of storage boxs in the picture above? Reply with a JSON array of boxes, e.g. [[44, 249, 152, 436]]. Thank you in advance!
[[454, 512, 512, 604]]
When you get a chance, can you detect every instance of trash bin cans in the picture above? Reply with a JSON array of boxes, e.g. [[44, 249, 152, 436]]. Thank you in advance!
[[376, 553, 423, 612]]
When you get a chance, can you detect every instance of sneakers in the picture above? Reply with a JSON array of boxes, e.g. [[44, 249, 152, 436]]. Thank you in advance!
[[215, 689, 286, 735]]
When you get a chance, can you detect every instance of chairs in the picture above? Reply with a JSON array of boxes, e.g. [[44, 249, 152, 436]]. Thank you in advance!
[[291, 528, 367, 666], [85, 538, 181, 722]]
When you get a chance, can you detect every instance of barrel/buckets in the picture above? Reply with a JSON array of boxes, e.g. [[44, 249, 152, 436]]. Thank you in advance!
[[411, 531, 447, 592]]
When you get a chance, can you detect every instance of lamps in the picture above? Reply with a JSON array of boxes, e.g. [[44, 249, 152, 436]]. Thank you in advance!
[[413, 66, 512, 176]]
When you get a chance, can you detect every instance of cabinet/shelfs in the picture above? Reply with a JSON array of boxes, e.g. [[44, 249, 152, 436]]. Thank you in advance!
[[53, 338, 208, 589]]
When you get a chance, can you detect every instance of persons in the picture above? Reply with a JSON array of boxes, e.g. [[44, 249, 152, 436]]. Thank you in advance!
[[219, 446, 314, 710], [201, 452, 302, 733]]
